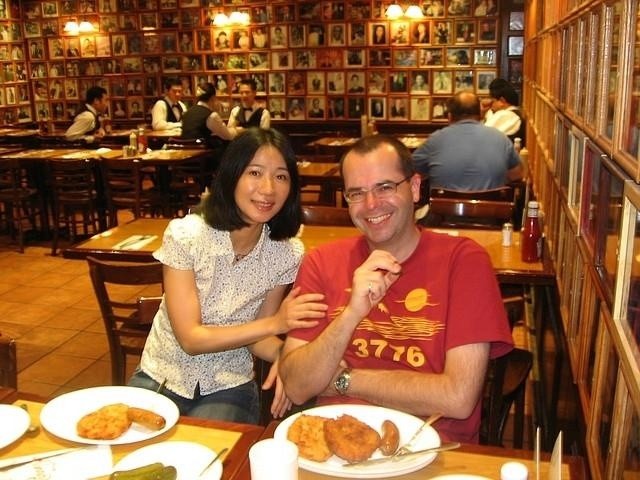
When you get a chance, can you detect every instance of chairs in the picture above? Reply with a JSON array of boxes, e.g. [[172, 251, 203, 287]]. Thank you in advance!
[[35, 136, 56, 142], [41, 143, 83, 149], [169, 138, 205, 144], [47, 158, 100, 255], [56, 138, 85, 144], [0, 158, 40, 253], [420, 177, 526, 231], [0, 143, 23, 148], [87, 256, 164, 386], [100, 157, 153, 227], [295, 175, 336, 207], [480, 297, 533, 449], [161, 144, 208, 150], [98, 144, 126, 150]]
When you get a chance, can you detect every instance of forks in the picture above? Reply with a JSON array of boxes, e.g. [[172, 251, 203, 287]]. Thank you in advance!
[[391, 410, 444, 463]]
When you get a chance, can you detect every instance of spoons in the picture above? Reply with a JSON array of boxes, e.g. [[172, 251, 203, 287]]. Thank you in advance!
[[20, 403, 39, 438]]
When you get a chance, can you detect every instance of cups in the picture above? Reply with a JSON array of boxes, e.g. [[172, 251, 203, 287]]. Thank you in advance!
[[248, 438, 299, 480]]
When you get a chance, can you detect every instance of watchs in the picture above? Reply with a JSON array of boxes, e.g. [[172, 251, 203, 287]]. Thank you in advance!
[[334, 368, 356, 399]]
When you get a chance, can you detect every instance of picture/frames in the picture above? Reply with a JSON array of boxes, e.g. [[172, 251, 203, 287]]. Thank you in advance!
[[507, 35, 525, 56], [508, 58, 522, 90], [508, 10, 526, 31]]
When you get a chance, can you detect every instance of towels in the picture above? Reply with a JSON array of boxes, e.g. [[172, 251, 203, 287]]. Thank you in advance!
[[0, 445, 113, 480], [112, 234, 158, 251]]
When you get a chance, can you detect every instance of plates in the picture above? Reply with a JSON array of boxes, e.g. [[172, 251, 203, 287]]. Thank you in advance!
[[274, 404, 440, 478], [40, 385, 180, 445], [430, 472, 498, 480], [112, 440, 223, 480], [0, 404, 31, 449]]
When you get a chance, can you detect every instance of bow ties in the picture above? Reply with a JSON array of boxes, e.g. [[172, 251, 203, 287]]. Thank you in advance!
[[171, 104, 178, 107], [243, 107, 253, 112]]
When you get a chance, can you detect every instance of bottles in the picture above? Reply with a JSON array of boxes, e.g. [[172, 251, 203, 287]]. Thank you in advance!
[[122, 145, 129, 158], [512, 138, 521, 157], [500, 462, 529, 480], [368, 116, 379, 137], [520, 201, 543, 263], [501, 223, 514, 247], [138, 128, 148, 155], [520, 148, 529, 181], [130, 132, 137, 151]]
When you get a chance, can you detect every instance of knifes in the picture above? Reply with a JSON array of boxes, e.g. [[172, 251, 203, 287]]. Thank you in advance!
[[341, 442, 460, 467]]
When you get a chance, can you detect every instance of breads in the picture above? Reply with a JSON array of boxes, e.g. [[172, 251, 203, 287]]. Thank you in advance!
[[287, 414, 335, 463], [75, 403, 132, 440], [324, 413, 380, 464]]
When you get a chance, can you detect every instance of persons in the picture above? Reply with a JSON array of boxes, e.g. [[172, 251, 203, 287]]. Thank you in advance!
[[0, 0, 499, 127], [410, 78, 525, 196], [61, 85, 110, 224], [279, 135, 515, 446], [150, 76, 271, 218], [127, 126, 328, 427]]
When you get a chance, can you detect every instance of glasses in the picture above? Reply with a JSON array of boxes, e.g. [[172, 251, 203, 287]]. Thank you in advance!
[[343, 177, 409, 203]]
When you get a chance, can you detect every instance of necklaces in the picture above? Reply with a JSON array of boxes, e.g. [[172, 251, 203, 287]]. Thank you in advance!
[[228, 228, 263, 262]]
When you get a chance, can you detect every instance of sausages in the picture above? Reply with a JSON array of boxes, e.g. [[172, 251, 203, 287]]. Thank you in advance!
[[380, 419, 399, 456], [126, 405, 166, 430]]
